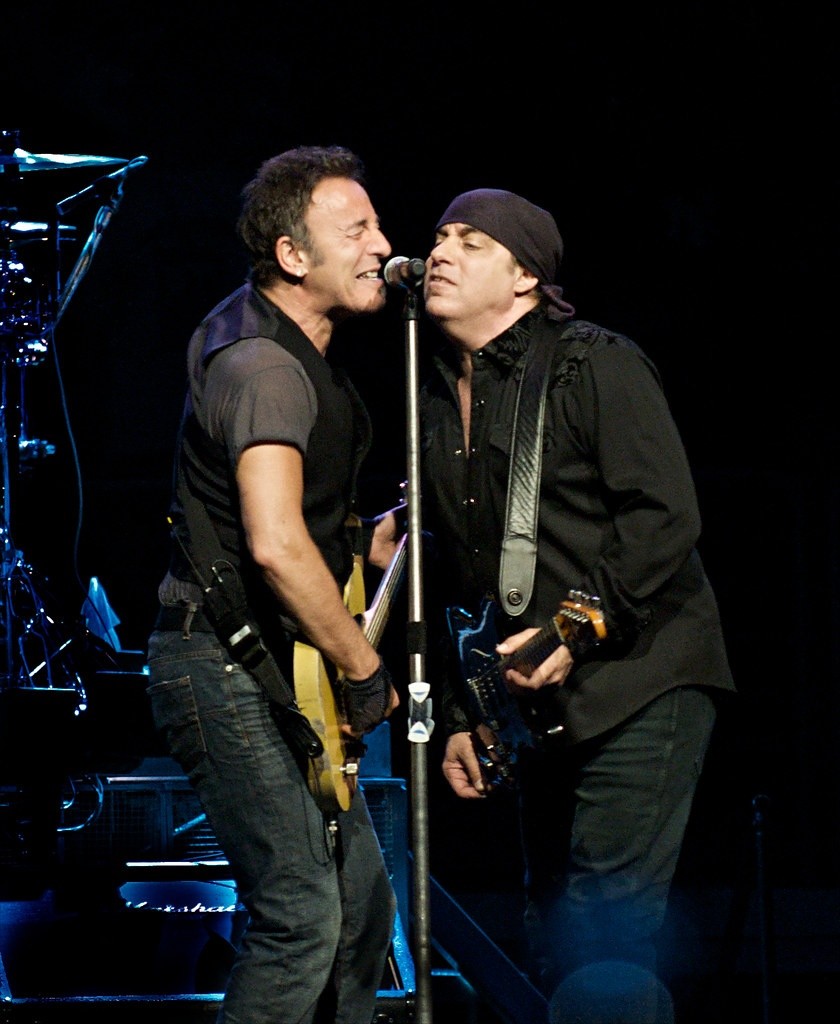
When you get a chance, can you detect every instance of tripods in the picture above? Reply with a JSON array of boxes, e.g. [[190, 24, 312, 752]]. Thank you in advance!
[[0, 168, 87, 706]]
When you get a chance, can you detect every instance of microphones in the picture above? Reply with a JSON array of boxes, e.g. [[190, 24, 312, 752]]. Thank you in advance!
[[383, 256, 426, 290], [56, 161, 147, 215]]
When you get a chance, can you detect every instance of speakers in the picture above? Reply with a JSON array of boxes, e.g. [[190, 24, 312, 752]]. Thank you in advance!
[[59, 777, 411, 947]]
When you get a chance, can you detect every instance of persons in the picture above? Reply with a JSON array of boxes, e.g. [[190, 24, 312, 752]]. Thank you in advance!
[[143, 146, 405, 1024], [418, 187, 738, 1024]]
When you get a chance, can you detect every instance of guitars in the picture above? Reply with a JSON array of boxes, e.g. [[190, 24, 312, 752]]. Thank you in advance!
[[293, 534, 409, 807], [433, 589, 607, 788]]
